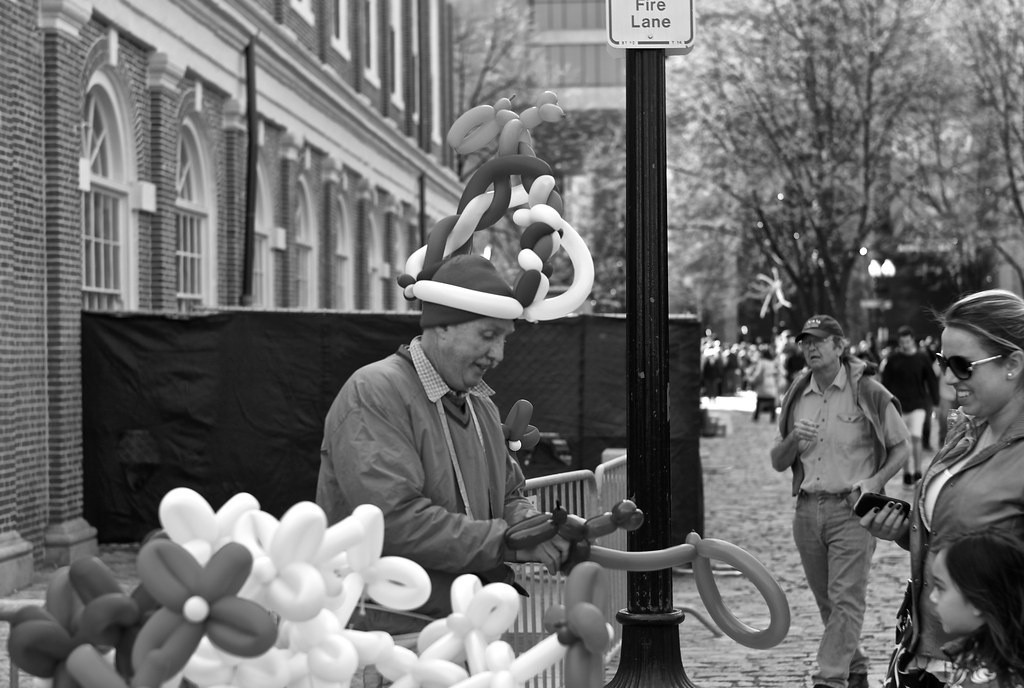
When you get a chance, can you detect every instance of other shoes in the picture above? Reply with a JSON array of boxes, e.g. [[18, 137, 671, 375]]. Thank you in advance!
[[904, 472, 912, 486], [814, 684, 831, 688], [913, 471, 922, 484], [846, 673, 868, 688]]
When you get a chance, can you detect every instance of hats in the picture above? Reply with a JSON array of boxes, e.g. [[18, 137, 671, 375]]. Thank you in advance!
[[795, 314, 844, 344], [419, 253, 514, 327]]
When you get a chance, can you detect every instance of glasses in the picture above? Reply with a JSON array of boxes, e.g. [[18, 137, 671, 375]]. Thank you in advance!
[[935, 351, 1013, 381], [797, 336, 837, 351]]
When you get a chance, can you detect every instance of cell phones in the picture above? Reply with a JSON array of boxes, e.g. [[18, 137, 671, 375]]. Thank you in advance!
[[853, 492, 910, 523]]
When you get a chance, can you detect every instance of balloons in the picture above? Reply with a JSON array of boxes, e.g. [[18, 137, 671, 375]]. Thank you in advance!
[[500, 399, 541, 454], [397, 89, 596, 323], [7, 487, 790, 688]]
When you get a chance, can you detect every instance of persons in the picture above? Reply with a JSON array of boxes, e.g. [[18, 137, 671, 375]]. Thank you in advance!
[[858, 287, 1024, 688], [929, 523, 1023, 688], [316, 253, 573, 637], [700, 326, 958, 489], [771, 314, 913, 688]]
